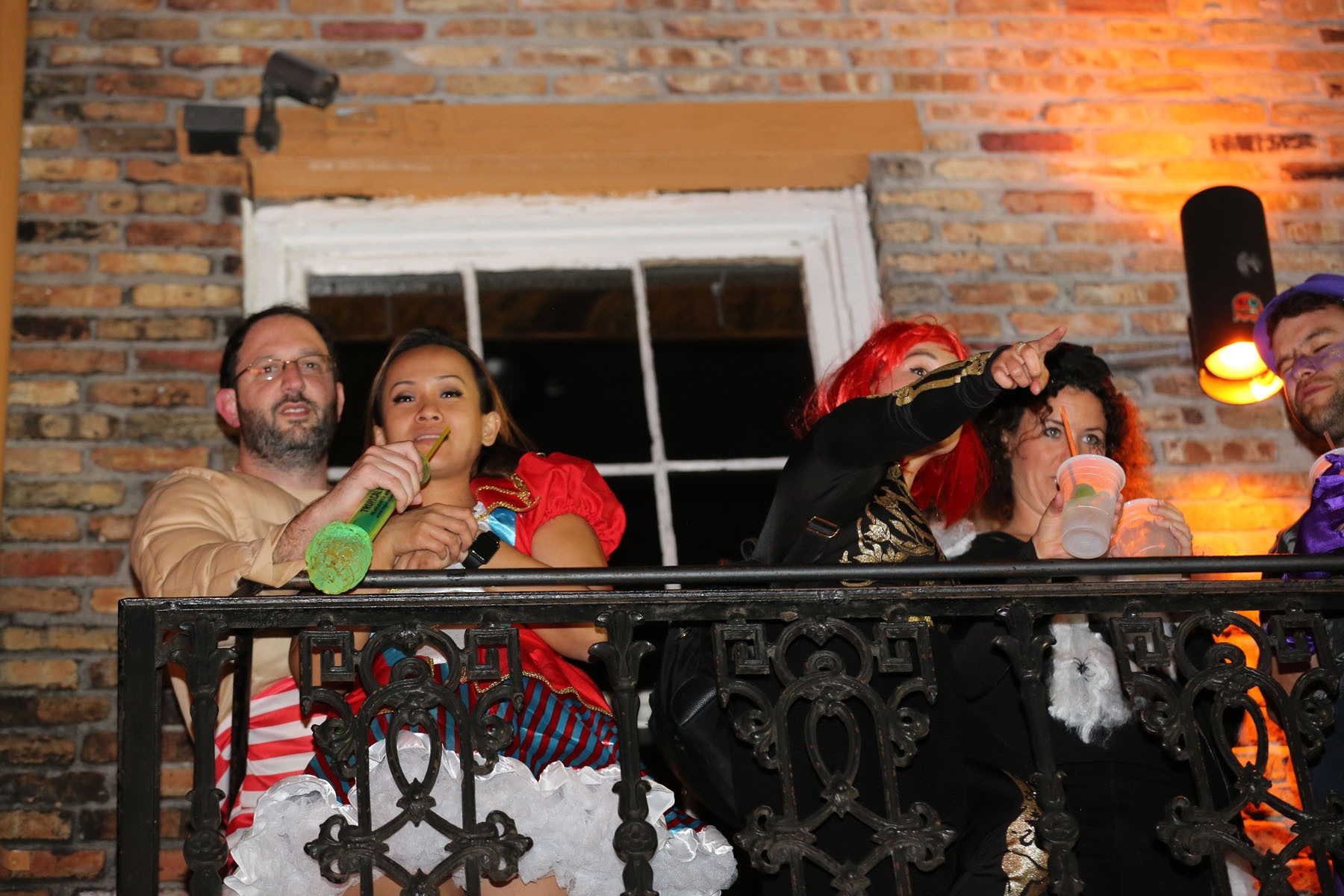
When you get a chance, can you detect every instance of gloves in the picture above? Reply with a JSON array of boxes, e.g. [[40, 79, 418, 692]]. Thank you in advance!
[[1311, 454, 1344, 538], [1266, 462, 1344, 655]]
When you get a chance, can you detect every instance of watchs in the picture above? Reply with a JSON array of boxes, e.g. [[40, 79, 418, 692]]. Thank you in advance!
[[461, 530, 501, 569]]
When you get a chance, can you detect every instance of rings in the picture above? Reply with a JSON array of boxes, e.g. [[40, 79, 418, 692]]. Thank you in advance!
[[1015, 342, 1027, 356]]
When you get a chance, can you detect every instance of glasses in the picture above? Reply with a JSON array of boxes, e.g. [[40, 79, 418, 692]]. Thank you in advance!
[[227, 354, 338, 387]]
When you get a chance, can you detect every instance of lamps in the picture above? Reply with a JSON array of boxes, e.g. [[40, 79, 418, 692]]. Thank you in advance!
[[1180, 186, 1285, 405]]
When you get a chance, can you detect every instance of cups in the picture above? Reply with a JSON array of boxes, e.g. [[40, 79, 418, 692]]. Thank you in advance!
[[1309, 447, 1344, 539], [1054, 453, 1126, 561], [1113, 497, 1183, 582]]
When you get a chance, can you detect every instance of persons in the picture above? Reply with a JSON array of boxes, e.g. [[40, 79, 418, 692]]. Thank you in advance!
[[1254, 272, 1344, 896], [122, 306, 423, 896], [908, 338, 1258, 894], [712, 317, 1069, 894], [217, 324, 738, 896]]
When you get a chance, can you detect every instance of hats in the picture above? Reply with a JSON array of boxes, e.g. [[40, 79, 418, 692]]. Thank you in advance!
[[1253, 273, 1344, 376]]
